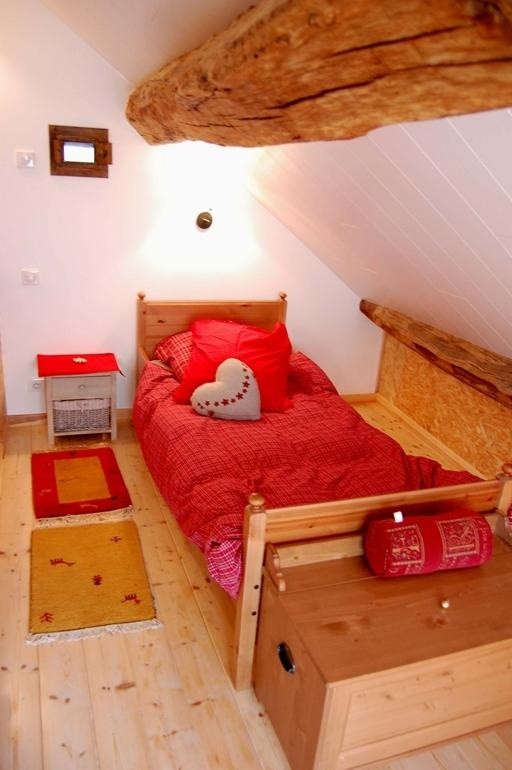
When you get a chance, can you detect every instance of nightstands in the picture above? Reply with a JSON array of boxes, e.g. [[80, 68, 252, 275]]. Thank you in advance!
[[37, 353, 123, 448]]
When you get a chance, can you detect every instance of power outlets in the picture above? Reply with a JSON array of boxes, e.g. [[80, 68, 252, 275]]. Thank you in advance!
[[28, 378, 44, 393]]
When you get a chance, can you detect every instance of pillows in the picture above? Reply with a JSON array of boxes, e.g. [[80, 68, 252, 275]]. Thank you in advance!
[[170, 314, 293, 414], [189, 358, 263, 424], [152, 332, 196, 382], [364, 511, 497, 580]]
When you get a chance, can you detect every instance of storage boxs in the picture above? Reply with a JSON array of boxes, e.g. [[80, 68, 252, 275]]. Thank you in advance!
[[251, 511, 511, 770]]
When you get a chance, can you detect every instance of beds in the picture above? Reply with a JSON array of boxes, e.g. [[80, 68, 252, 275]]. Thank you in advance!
[[130, 285, 512, 692]]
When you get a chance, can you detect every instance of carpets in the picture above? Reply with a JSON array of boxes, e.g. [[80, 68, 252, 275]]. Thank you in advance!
[[26, 520, 157, 637], [30, 445, 134, 520]]
